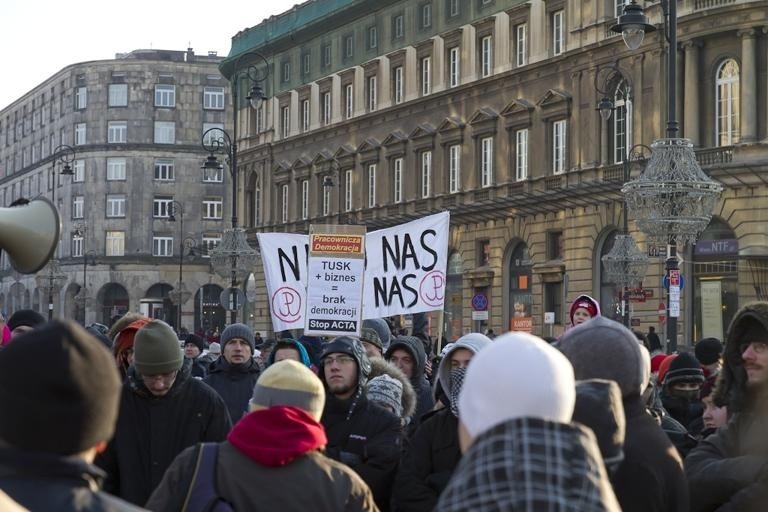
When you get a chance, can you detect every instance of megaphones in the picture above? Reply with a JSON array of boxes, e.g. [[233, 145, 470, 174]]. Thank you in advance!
[[0, 196, 60, 275]]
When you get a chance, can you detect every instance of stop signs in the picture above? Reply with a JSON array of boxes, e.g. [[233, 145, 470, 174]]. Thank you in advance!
[[657, 302, 666, 323]]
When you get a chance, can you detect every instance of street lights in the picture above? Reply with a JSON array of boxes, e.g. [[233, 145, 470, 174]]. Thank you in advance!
[[592, 65, 653, 331], [199, 50, 270, 325], [165, 200, 196, 338], [35, 144, 76, 324], [610, 1, 725, 358], [72, 217, 97, 329], [319, 156, 359, 227]]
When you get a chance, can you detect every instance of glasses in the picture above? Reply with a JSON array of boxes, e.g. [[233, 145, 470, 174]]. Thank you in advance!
[[739, 343, 767, 354], [319, 356, 356, 367]]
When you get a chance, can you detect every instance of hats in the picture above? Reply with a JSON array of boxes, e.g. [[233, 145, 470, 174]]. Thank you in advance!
[[570, 298, 597, 319], [694, 337, 723, 365], [184, 333, 204, 353], [1, 307, 122, 459], [220, 323, 256, 356], [652, 351, 705, 387], [133, 319, 184, 376], [365, 373, 403, 418], [248, 357, 327, 426], [555, 317, 653, 471], [357, 314, 392, 352], [457, 330, 577, 443], [319, 335, 371, 390]]
